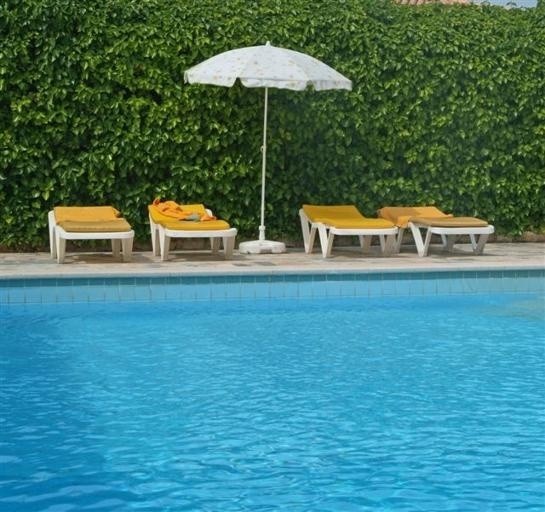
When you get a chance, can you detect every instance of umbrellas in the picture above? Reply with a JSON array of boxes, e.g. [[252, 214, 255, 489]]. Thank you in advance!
[[183, 39, 353, 227]]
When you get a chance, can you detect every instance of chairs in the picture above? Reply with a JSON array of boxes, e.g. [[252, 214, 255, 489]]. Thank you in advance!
[[377, 206, 495, 258], [147, 198, 238, 260], [298, 204, 399, 259], [48, 206, 136, 264]]
